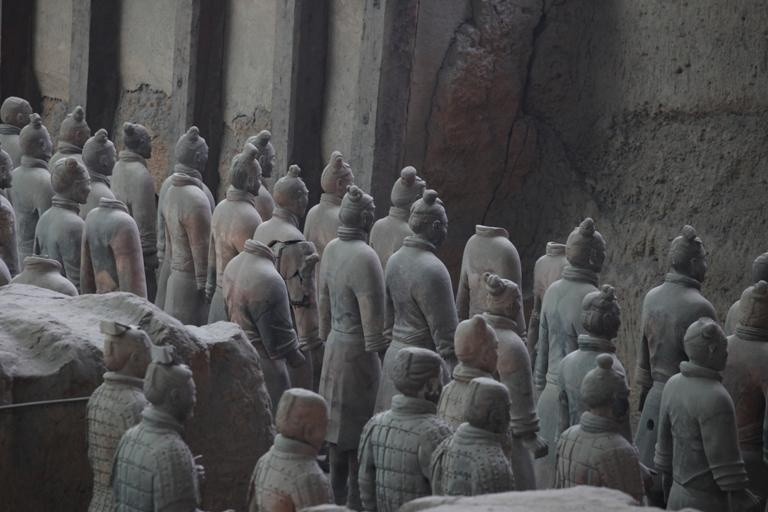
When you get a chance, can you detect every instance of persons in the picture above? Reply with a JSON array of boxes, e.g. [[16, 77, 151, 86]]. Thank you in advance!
[[1, 96, 457, 418], [87, 218, 768, 512]]
